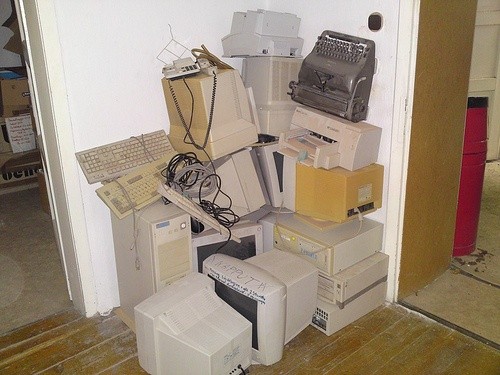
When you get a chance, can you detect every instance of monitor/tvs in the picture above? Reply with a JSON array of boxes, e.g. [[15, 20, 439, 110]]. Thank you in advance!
[[191, 220, 263, 273], [204, 248, 320, 367], [255, 142, 283, 208], [133, 272, 252, 375], [284, 153, 383, 224], [203, 147, 270, 226]]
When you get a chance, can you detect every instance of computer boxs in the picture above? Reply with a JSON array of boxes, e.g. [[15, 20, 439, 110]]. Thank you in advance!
[[110, 198, 191, 321], [239, 55, 303, 139]]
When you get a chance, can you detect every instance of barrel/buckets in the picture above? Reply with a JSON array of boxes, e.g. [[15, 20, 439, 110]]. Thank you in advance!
[[452, 92, 489, 257]]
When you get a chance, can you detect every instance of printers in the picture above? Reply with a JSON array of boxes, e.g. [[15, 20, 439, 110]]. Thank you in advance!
[[278, 104, 382, 171]]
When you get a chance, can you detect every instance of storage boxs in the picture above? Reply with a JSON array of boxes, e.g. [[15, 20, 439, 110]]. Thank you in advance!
[[1, 78, 30, 105]]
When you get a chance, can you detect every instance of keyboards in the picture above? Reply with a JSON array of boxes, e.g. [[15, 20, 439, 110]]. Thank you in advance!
[[95, 150, 188, 220], [75, 129, 174, 184], [158, 180, 241, 243]]
